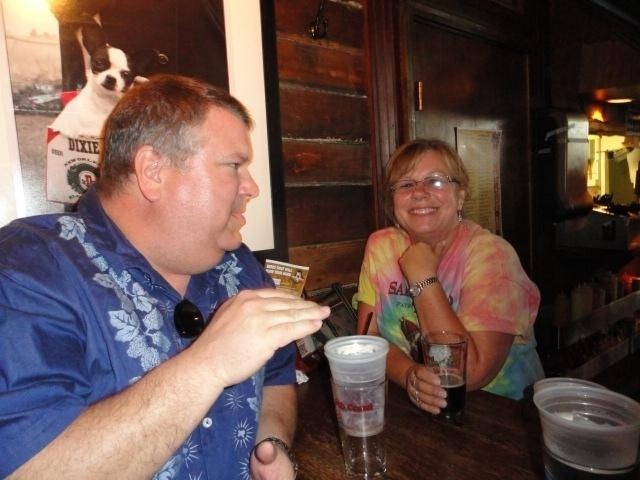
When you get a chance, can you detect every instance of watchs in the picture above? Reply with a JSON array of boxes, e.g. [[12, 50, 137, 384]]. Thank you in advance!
[[251, 438, 303, 475], [405, 276, 442, 295]]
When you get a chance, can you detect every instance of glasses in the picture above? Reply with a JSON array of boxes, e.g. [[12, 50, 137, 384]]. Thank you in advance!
[[173, 300, 206, 339], [390, 176, 461, 195]]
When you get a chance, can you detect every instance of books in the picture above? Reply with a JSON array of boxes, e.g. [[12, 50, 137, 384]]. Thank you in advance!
[[262, 259, 309, 300]]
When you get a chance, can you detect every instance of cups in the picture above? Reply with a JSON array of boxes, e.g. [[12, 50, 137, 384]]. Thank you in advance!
[[419, 330, 468, 427], [323, 333, 392, 438], [532, 383, 640, 480], [329, 375, 388, 480]]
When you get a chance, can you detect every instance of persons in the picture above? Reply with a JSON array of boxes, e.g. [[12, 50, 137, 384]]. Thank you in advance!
[[0, 74, 331, 480], [355, 139, 547, 414]]
